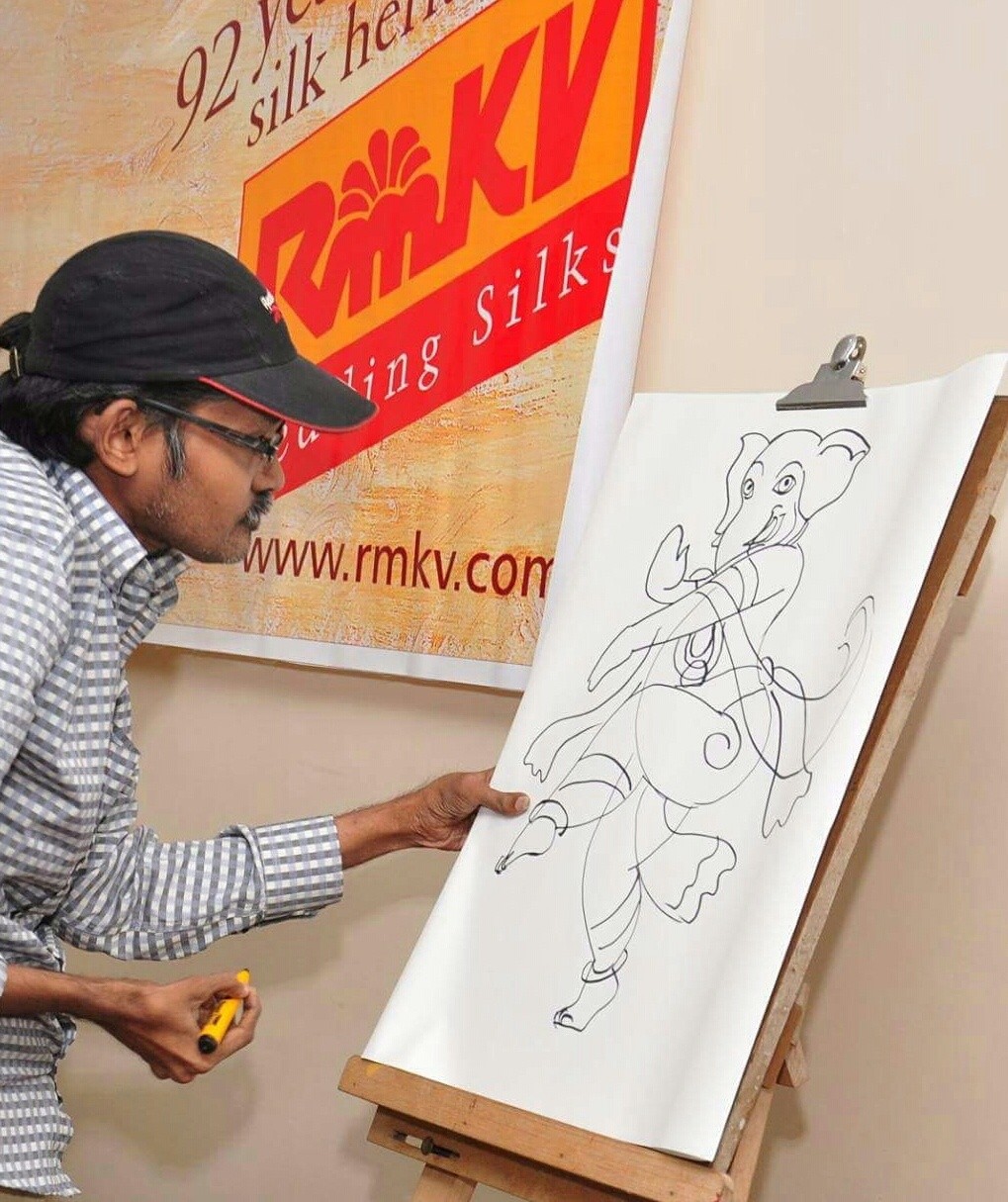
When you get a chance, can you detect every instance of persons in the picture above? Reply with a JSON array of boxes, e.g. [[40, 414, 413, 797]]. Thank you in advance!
[[0, 228, 531, 1202]]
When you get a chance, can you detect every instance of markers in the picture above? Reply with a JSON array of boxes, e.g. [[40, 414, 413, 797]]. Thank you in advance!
[[193, 970, 252, 1054]]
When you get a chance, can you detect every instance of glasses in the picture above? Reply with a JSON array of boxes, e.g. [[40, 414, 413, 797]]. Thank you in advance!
[[92, 390, 288, 463]]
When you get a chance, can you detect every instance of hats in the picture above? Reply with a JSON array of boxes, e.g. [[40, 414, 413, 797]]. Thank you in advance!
[[0, 231, 382, 434]]
